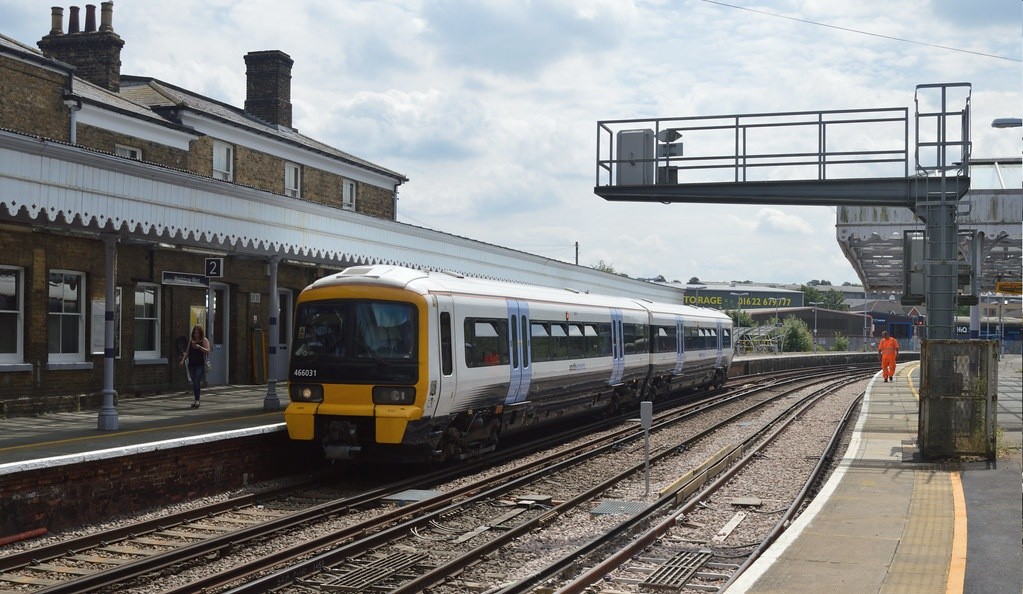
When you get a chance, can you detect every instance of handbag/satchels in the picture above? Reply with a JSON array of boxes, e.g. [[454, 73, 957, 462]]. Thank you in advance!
[[204, 359, 212, 373]]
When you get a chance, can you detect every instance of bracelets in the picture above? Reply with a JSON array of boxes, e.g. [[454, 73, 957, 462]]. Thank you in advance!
[[199, 347, 201, 349]]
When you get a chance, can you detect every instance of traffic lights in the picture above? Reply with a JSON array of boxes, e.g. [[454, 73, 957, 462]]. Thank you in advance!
[[918, 316, 924, 325]]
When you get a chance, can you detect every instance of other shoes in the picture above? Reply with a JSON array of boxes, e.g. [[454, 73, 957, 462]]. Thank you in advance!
[[884, 378, 888, 383], [889, 376, 893, 381], [191, 402, 200, 407], [194, 401, 200, 409]]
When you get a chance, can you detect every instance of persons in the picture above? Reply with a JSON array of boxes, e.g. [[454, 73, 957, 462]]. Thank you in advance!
[[878, 331, 899, 382], [365, 313, 401, 353], [180, 326, 210, 408]]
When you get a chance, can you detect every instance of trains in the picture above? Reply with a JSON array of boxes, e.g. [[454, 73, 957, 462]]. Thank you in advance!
[[284, 262, 735, 479], [954, 316, 1023, 353]]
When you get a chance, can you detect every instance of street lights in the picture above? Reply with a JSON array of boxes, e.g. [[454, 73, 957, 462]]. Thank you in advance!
[[687, 285, 707, 306], [769, 297, 786, 354], [809, 302, 824, 353], [991, 300, 1008, 350], [730, 292, 749, 356]]
